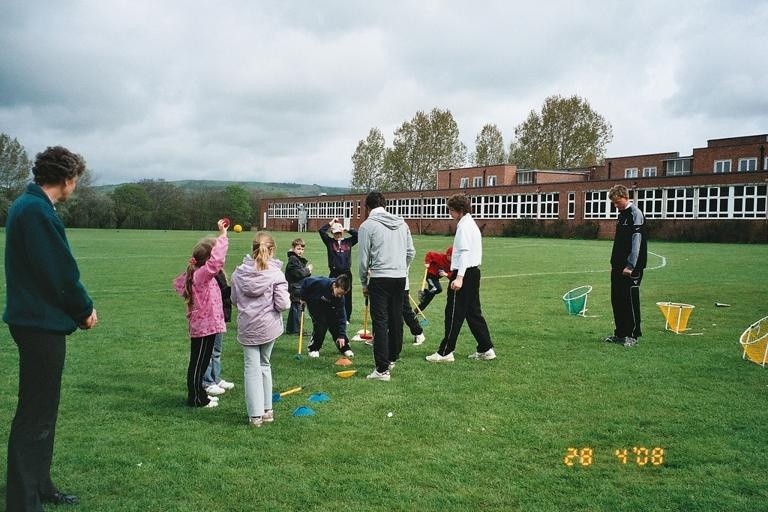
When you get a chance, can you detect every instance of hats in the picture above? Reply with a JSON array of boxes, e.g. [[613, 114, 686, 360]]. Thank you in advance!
[[330, 223, 343, 233]]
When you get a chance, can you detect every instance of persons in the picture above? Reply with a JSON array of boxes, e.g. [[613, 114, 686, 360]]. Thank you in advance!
[[172, 219, 230, 409], [285, 218, 358, 359], [605, 184, 647, 347], [230, 231, 292, 427], [199, 237, 234, 395], [414, 246, 453, 316], [358, 191, 416, 382], [365, 264, 426, 346], [1, 145, 98, 512], [426, 193, 496, 362]]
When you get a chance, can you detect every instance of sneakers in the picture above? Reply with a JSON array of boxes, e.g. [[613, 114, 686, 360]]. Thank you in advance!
[[344, 350, 353, 356], [249, 410, 274, 424], [413, 332, 425, 345], [205, 380, 234, 395], [603, 336, 619, 342], [308, 351, 319, 358], [624, 336, 637, 346], [468, 348, 496, 360], [204, 395, 219, 407], [367, 370, 390, 380], [425, 352, 454, 362], [40, 493, 78, 504]]
